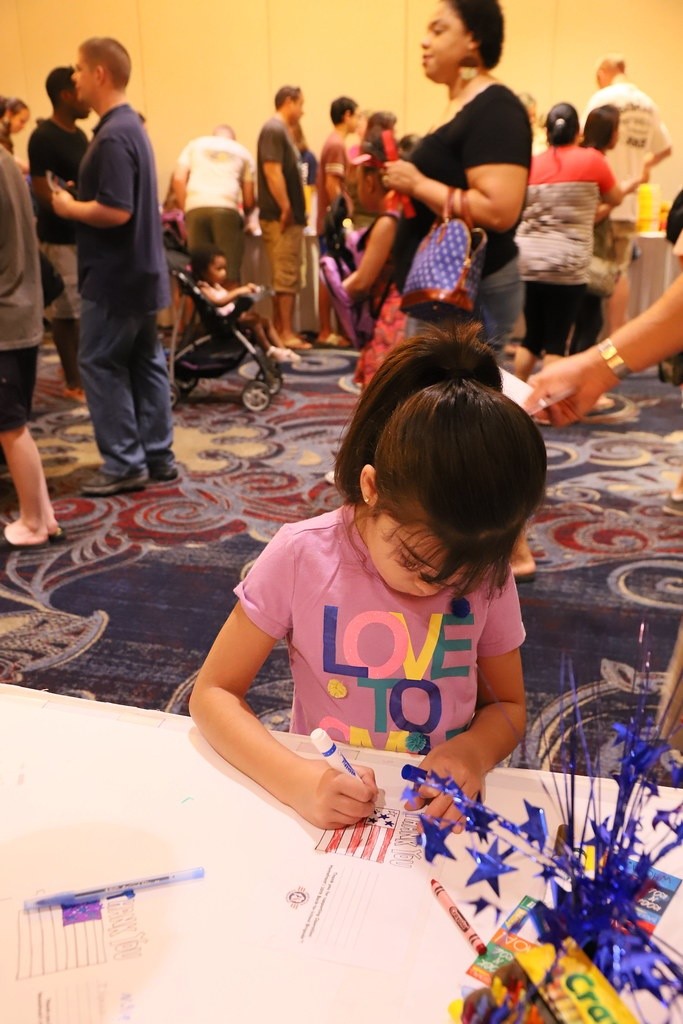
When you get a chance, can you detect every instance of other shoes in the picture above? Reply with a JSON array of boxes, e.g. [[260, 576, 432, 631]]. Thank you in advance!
[[338, 374, 362, 395], [267, 346, 300, 364], [282, 333, 312, 350], [316, 334, 350, 347]]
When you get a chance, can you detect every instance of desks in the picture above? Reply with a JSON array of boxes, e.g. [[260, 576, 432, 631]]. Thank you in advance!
[[0, 676, 683, 1024]]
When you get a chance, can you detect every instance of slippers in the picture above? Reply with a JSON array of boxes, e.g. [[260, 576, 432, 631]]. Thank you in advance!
[[0, 523, 68, 552]]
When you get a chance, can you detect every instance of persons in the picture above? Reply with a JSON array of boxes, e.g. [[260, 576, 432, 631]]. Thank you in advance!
[[188, 328, 549, 834], [1, 1, 683, 584]]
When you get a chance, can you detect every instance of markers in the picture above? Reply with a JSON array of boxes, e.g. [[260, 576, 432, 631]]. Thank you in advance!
[[310, 728, 365, 784], [401, 764, 427, 782]]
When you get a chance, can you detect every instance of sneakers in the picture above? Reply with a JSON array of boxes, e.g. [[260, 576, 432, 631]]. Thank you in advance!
[[79, 463, 178, 494]]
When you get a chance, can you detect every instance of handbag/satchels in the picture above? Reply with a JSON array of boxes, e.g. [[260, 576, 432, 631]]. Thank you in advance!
[[400, 187, 489, 321]]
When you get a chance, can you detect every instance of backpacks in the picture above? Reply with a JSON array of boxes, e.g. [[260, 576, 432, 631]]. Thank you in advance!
[[323, 209, 402, 350]]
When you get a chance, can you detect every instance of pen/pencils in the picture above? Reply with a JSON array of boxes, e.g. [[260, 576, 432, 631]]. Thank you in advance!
[[430, 879, 487, 955], [23, 866, 204, 909]]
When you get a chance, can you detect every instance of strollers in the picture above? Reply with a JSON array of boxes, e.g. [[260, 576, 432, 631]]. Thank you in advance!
[[160, 208, 284, 412]]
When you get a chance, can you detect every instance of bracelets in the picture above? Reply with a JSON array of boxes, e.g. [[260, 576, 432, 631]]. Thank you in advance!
[[598, 335, 635, 381]]
